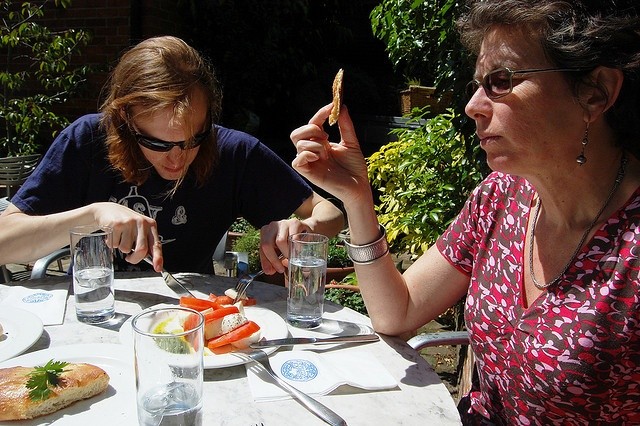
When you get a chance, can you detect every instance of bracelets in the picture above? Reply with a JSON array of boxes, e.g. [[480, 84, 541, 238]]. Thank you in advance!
[[342, 224, 391, 266]]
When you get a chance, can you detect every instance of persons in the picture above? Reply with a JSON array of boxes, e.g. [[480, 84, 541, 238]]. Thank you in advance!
[[0, 34, 345, 276], [290, 1, 640, 426]]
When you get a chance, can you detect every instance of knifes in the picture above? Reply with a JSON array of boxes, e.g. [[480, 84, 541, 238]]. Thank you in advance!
[[250, 330, 380, 350], [118, 232, 198, 301]]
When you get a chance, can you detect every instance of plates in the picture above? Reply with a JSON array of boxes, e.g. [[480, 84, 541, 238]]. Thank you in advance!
[[1, 343, 137, 426], [1, 301, 44, 360], [120, 303, 288, 369]]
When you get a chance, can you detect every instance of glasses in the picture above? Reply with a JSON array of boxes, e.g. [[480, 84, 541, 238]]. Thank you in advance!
[[127, 112, 212, 151], [466, 67, 594, 99]]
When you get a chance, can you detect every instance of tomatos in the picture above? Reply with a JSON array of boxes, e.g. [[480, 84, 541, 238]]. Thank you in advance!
[[205, 306, 240, 322], [209, 294, 256, 306], [179, 296, 215, 309], [208, 322, 260, 349]]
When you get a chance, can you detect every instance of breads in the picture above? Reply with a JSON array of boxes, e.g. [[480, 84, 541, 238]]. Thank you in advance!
[[1, 363, 110, 421]]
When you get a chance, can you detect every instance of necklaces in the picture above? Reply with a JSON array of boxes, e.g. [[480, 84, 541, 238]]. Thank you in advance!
[[529, 150, 626, 289]]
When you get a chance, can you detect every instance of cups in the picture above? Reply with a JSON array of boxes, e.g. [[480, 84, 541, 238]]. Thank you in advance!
[[286, 233, 329, 328], [69, 224, 117, 324], [131, 308, 205, 425]]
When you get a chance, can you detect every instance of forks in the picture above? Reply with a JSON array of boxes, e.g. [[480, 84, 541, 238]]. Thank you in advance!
[[243, 347, 345, 426], [234, 230, 308, 302]]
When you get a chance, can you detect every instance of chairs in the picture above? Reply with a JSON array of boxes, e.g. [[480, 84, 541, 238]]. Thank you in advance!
[[405, 332, 474, 400], [30, 229, 249, 282], [0, 154, 42, 211]]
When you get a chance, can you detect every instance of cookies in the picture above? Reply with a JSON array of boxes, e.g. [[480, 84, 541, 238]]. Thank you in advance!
[[329, 68, 344, 126]]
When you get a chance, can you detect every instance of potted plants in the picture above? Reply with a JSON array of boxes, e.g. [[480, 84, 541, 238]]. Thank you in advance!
[[282, 243, 356, 295], [290, 283, 372, 317]]
